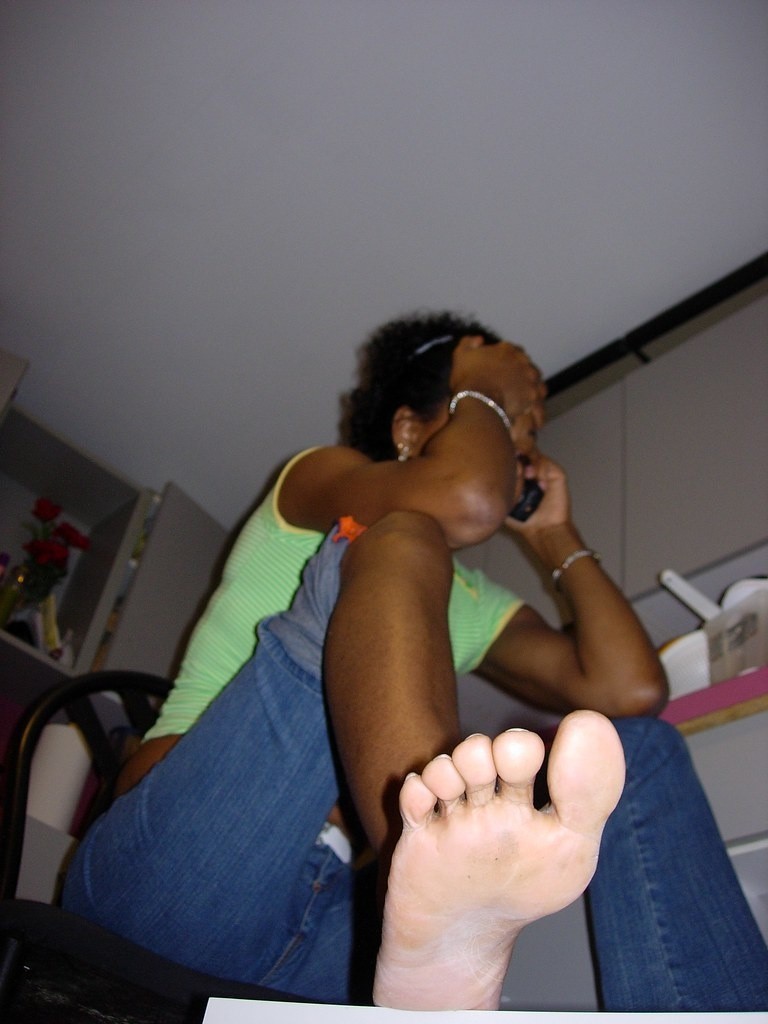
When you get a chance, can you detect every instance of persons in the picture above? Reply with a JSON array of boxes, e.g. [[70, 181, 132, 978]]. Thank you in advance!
[[61, 315, 768, 1011]]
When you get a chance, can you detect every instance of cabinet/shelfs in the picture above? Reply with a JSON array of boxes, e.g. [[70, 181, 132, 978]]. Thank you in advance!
[[437, 286, 768, 633]]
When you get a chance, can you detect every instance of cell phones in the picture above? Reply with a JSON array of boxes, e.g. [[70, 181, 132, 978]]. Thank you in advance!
[[508, 453, 545, 522]]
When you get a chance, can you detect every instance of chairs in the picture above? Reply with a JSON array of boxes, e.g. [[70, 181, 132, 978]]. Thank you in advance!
[[0, 671, 325, 1023]]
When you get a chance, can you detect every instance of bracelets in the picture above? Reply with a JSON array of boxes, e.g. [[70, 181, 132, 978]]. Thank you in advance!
[[551, 549, 602, 591], [449, 390, 510, 429]]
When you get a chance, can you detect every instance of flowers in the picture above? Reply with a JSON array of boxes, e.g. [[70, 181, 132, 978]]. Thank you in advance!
[[14, 496, 99, 627]]
